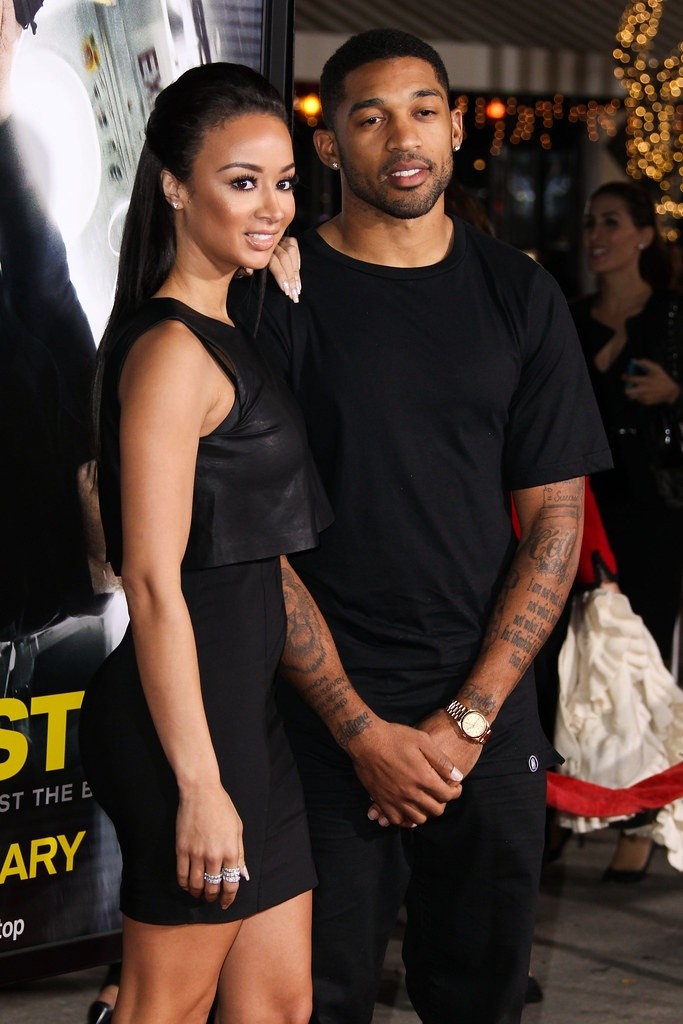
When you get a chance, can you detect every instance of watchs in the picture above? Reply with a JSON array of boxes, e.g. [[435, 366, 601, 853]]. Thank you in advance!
[[441, 700, 492, 745]]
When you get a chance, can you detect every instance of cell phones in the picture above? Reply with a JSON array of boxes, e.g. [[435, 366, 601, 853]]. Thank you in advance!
[[627, 358, 648, 389]]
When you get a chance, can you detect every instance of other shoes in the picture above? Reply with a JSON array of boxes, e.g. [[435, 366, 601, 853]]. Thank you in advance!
[[601, 838, 655, 888]]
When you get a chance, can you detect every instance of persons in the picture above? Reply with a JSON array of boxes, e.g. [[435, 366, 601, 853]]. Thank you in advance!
[[77, 30, 683, 1024]]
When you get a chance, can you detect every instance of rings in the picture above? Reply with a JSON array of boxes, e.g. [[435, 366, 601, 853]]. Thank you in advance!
[[222, 867, 241, 883], [204, 873, 222, 885]]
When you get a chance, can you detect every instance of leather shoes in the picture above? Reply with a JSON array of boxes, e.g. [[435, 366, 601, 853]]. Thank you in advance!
[[87, 1000, 112, 1024]]
